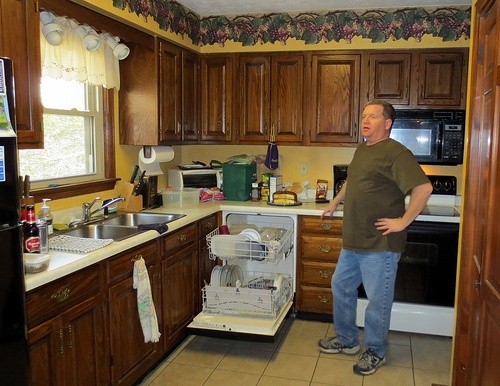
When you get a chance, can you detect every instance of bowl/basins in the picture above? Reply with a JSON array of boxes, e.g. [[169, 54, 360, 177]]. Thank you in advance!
[[24, 253, 50, 274], [210, 222, 296, 266]]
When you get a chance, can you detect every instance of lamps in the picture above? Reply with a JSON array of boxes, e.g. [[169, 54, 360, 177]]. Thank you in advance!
[[104, 33, 130, 60], [76, 24, 101, 52], [39, 11, 64, 46]]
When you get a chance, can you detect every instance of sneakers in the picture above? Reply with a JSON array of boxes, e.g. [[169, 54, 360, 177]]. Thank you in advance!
[[353, 348, 386, 375], [318, 336, 361, 355]]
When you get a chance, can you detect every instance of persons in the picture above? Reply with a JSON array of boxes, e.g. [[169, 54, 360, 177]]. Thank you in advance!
[[317, 101, 433, 376]]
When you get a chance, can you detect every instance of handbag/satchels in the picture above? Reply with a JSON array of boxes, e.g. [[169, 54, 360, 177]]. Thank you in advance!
[[223, 158, 257, 202], [264, 141, 279, 170]]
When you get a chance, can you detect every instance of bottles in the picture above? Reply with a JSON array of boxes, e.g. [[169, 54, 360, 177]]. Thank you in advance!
[[21, 196, 40, 255], [250, 183, 261, 202]]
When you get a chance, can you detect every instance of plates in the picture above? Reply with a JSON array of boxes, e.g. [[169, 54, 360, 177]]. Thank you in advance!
[[210, 264, 287, 302]]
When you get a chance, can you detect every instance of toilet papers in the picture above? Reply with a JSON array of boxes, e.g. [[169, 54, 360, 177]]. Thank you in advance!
[[138, 146, 174, 177]]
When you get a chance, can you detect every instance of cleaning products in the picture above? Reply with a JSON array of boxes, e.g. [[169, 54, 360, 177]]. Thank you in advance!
[[38, 198, 53, 236]]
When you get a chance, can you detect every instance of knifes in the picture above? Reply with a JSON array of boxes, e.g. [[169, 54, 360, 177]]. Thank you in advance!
[[131, 165, 146, 196]]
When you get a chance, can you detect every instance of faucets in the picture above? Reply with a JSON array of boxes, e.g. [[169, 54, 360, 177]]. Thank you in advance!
[[68, 196, 126, 230], [103, 196, 120, 219]]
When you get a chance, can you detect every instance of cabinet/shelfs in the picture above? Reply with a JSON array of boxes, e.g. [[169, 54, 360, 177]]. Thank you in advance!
[[26, 211, 223, 386], [120, 37, 470, 147], [295, 217, 345, 322], [0, 0, 44, 149]]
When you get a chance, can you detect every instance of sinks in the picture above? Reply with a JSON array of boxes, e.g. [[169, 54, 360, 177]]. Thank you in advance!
[[102, 212, 187, 226], [59, 226, 145, 242]]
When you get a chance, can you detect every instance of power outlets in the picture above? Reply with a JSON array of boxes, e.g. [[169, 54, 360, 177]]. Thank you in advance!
[[300, 161, 307, 174]]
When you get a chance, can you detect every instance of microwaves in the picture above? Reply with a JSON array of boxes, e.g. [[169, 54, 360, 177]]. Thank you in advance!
[[166, 167, 224, 191]]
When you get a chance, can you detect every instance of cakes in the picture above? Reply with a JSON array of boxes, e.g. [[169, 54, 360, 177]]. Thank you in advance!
[[272, 191, 297, 205]]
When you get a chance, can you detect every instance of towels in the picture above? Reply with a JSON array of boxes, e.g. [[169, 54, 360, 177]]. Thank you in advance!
[[132, 257, 162, 344]]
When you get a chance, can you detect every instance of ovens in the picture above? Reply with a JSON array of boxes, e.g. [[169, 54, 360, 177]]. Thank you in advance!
[[351, 218, 461, 338], [364, 109, 466, 165]]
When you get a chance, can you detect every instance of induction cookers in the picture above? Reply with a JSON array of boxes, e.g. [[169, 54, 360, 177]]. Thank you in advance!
[[402, 175, 464, 223]]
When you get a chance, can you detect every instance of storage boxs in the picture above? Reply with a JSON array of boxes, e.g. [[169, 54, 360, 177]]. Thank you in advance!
[[222, 161, 257, 201]]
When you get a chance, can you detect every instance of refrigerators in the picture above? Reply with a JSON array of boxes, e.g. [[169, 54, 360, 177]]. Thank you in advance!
[[0, 57, 32, 386]]
